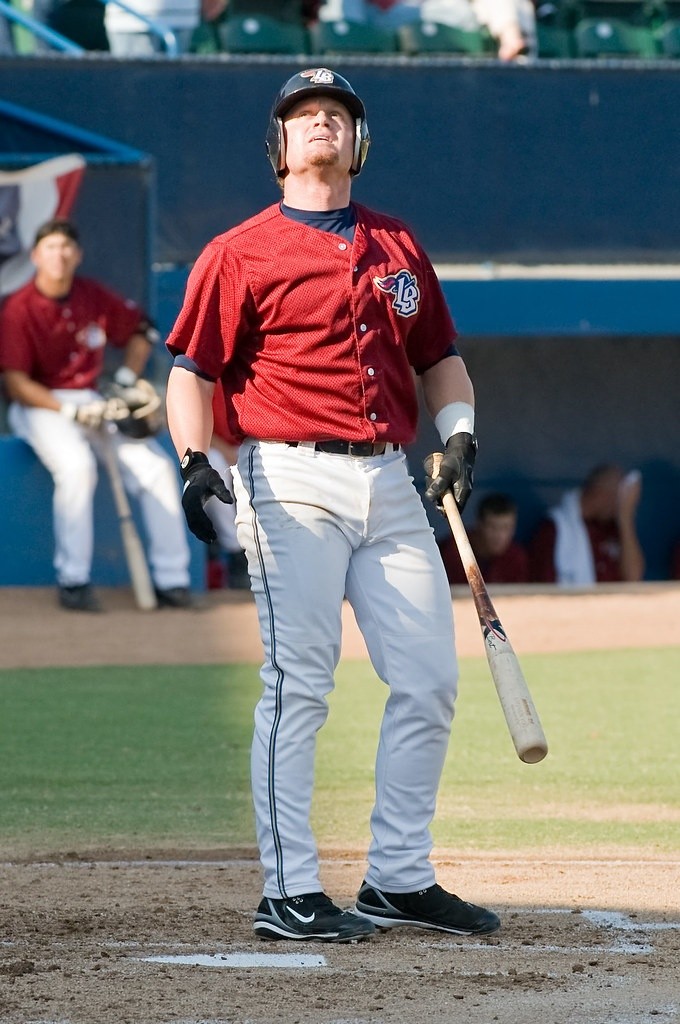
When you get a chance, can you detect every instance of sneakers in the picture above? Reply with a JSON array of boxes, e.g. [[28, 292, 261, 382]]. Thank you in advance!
[[354, 878, 501, 935], [253, 891, 376, 942]]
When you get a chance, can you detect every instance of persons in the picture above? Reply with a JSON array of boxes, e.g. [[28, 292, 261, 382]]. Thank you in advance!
[[204, 437, 251, 589], [0, 221, 192, 612], [165, 68, 500, 941], [35, 0, 538, 60], [436, 464, 642, 587]]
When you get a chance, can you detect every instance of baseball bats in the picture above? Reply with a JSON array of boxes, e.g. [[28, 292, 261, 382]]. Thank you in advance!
[[421, 451, 549, 766], [100, 426, 158, 610]]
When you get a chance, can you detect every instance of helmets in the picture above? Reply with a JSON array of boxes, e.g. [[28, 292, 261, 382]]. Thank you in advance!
[[263, 67, 370, 177]]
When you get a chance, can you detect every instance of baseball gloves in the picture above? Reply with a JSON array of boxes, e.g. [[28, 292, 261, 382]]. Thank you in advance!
[[108, 378, 164, 440]]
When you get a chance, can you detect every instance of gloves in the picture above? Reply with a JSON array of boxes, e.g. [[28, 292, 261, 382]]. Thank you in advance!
[[73, 398, 107, 431], [180, 448, 233, 542], [424, 431, 478, 518]]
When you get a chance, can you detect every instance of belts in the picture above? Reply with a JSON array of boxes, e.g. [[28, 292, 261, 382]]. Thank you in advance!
[[285, 438, 400, 457]]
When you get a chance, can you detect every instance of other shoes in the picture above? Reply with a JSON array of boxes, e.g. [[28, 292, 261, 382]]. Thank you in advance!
[[153, 585, 192, 610], [58, 583, 104, 615]]
